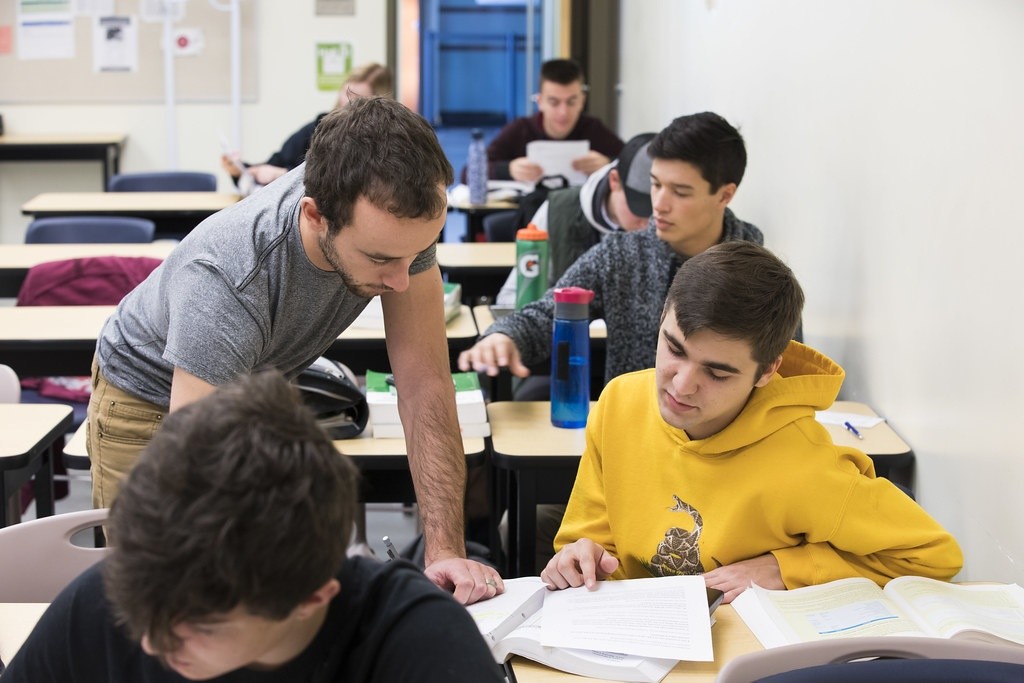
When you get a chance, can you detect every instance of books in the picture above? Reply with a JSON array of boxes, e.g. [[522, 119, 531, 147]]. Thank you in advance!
[[349, 282, 461, 327], [365, 368, 491, 438], [458, 575, 724, 683], [731, 576, 1024, 663]]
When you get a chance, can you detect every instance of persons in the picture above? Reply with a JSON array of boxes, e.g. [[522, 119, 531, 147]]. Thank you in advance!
[[458, 61, 807, 580], [0, 371, 505, 683], [85, 95, 505, 606], [542, 241, 964, 605], [219, 64, 398, 189]]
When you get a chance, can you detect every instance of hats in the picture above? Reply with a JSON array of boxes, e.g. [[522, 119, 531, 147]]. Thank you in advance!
[[618, 133, 659, 216]]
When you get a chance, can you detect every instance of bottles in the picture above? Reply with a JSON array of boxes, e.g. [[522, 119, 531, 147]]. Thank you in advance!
[[550, 286, 589, 429], [468, 130, 488, 205], [516, 225, 550, 314]]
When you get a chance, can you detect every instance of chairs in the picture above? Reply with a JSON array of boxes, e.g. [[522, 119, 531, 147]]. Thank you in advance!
[[109, 173, 217, 242], [5, 256, 163, 428], [25, 217, 156, 247], [0, 365, 20, 406], [484, 212, 522, 299], [718, 637, 1024, 683], [0, 508, 111, 603]]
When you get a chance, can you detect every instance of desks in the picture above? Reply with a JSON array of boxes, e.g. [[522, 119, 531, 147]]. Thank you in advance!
[[0, 131, 1024, 683]]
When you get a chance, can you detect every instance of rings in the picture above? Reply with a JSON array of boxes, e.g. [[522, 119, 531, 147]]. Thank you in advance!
[[486, 578, 497, 585]]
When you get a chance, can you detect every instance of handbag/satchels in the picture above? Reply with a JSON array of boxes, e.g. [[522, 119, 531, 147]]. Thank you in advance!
[[290, 356, 369, 439]]
